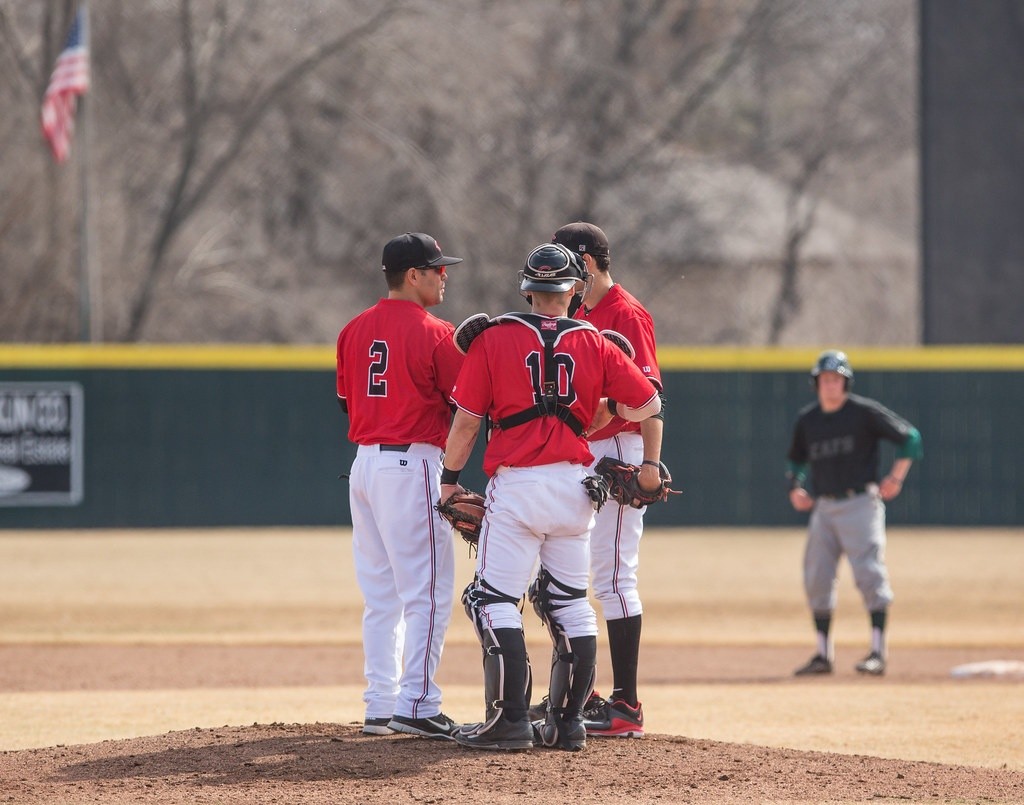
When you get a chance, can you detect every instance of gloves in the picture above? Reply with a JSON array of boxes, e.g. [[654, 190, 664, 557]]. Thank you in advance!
[[581, 475, 610, 513]]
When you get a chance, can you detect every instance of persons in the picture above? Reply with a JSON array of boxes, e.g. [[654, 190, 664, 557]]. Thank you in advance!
[[433, 243, 661, 752], [788, 351, 920, 676], [336, 232, 464, 738], [530, 220, 670, 738]]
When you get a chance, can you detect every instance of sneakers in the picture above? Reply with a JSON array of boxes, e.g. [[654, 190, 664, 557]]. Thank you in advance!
[[529, 691, 610, 729], [387, 712, 456, 740], [532, 717, 586, 750], [362, 716, 401, 735], [455, 714, 533, 749], [582, 697, 644, 738]]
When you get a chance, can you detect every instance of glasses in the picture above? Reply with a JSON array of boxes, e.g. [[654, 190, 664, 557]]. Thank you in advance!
[[403, 265, 446, 276]]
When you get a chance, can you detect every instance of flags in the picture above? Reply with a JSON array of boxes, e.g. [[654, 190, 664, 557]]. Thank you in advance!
[[42, 10, 89, 161]]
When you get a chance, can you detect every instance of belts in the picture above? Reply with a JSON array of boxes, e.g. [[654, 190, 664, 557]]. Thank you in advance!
[[822, 486, 866, 500], [380, 444, 411, 452]]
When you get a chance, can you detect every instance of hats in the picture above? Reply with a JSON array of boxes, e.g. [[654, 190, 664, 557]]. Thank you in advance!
[[382, 232, 463, 271], [551, 220, 609, 257]]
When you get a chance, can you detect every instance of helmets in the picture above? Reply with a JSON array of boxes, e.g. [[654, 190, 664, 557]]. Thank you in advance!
[[811, 350, 852, 393], [520, 247, 578, 292]]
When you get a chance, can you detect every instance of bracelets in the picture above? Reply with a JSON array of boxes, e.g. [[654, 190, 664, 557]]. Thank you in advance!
[[607, 399, 618, 416], [441, 466, 461, 486], [889, 474, 904, 485], [642, 461, 659, 468]]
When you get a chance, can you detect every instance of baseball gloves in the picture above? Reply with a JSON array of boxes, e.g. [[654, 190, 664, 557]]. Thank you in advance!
[[581, 472, 613, 514], [594, 455, 672, 509], [437, 490, 485, 546]]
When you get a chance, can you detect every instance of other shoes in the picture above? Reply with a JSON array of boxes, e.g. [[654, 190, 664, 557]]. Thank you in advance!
[[858, 655, 885, 675], [796, 659, 833, 676]]
